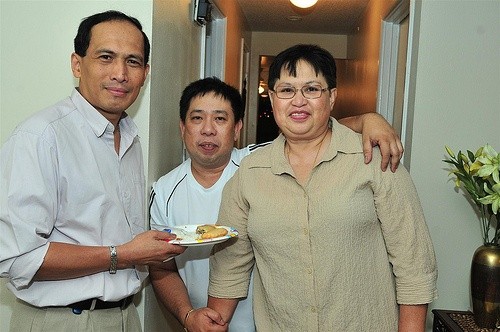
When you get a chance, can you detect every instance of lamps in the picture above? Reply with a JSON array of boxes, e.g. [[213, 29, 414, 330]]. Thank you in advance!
[[290, 0, 318, 9]]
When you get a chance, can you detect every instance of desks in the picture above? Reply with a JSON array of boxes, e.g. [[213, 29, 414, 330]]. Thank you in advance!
[[432, 309, 500, 332]]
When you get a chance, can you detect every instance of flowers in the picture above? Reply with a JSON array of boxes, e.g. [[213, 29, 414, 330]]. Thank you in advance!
[[442, 142, 500, 244]]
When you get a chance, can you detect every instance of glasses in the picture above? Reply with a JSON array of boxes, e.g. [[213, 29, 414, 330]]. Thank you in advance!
[[273, 85, 330, 99]]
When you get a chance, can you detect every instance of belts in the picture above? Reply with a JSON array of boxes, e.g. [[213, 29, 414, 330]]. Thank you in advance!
[[67, 294, 134, 310]]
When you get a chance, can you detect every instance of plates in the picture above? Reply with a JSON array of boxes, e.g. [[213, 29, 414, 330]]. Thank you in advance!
[[161, 224, 239, 246]]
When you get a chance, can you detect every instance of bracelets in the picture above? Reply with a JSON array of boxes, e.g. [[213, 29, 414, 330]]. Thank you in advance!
[[184, 309, 195, 332], [108, 246, 118, 275]]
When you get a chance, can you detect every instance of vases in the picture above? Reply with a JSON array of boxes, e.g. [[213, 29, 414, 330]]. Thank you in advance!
[[471, 243, 500, 328]]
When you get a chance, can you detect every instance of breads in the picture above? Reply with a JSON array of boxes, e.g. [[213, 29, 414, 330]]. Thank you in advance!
[[196, 225, 227, 239]]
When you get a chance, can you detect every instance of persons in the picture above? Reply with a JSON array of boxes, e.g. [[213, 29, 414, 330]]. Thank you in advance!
[[0, 10, 188, 332], [206, 44, 438, 332], [148, 77, 404, 332]]
